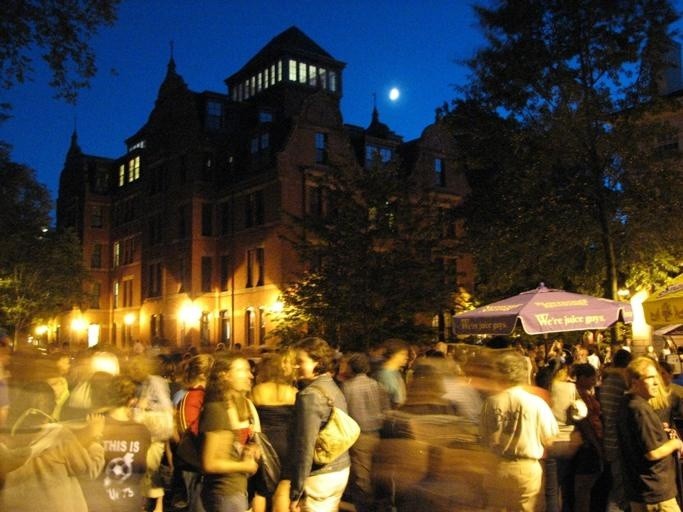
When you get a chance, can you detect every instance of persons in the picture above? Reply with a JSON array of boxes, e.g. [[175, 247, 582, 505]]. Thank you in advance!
[[2, 330, 682, 512]]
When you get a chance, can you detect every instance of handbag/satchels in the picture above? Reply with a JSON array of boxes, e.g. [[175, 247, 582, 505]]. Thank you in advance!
[[313, 407, 361, 466], [173, 428, 201, 472], [254, 434, 281, 495]]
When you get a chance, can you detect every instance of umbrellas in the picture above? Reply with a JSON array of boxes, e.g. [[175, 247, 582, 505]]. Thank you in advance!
[[453, 282, 634, 344], [642, 272, 683, 328]]
